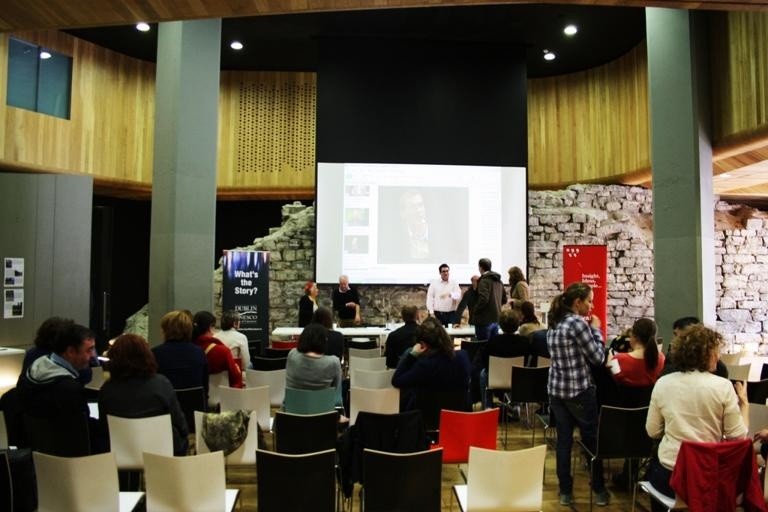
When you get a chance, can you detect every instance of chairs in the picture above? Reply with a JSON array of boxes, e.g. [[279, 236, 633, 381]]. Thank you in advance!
[[0, 322, 767, 512]]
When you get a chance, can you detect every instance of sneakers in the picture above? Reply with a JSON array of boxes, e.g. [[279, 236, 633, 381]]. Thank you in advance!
[[559, 495, 574, 505], [594, 491, 613, 506]]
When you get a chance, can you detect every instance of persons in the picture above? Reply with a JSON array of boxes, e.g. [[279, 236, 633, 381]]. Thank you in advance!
[[97, 334, 188, 457], [606, 316, 666, 488], [148, 309, 252, 400], [332, 276, 361, 326], [298, 282, 319, 328], [425, 258, 548, 404], [546, 282, 613, 506], [752, 425, 768, 462], [16, 327, 110, 455], [384, 306, 472, 404], [285, 309, 350, 406], [673, 317, 729, 379], [645, 324, 750, 511], [21, 317, 74, 362]]
